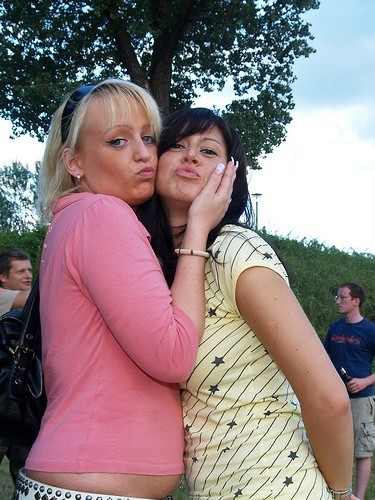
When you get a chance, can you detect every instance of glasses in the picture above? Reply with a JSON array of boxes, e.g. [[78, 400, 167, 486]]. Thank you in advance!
[[334, 295, 350, 301]]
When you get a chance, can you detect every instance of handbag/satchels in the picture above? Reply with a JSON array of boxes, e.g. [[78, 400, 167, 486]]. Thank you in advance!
[[0, 311, 48, 466]]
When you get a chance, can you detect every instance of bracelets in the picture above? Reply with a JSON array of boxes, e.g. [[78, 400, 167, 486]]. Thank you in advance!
[[174, 248, 210, 259], [329, 487, 353, 500]]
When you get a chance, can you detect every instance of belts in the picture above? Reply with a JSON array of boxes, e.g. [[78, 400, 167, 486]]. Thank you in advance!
[[14, 470, 152, 500]]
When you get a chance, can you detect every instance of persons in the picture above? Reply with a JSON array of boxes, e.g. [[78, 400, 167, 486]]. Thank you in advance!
[[0, 247, 34, 318], [132, 108, 358, 500], [323, 282, 375, 500], [12, 78, 239, 500]]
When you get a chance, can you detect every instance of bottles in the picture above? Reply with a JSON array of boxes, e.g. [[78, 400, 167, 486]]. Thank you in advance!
[[339, 366, 352, 384]]
